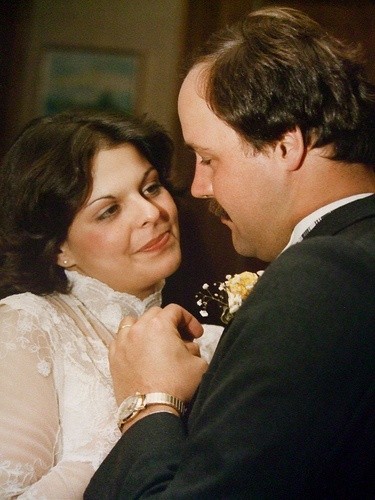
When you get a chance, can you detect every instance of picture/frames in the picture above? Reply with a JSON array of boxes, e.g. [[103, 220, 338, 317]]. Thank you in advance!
[[33, 39, 151, 121]]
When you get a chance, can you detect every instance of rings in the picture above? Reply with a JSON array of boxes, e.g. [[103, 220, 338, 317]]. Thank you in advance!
[[117, 325, 132, 332]]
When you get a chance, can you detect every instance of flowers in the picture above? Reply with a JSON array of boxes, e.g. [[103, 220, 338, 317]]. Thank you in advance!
[[193, 265, 266, 324]]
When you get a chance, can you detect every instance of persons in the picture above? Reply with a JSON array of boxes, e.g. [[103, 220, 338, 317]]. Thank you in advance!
[[0, 106, 226, 500], [81, 4, 375, 500]]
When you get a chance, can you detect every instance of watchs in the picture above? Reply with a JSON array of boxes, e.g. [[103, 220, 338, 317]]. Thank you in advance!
[[116, 390, 188, 432]]
